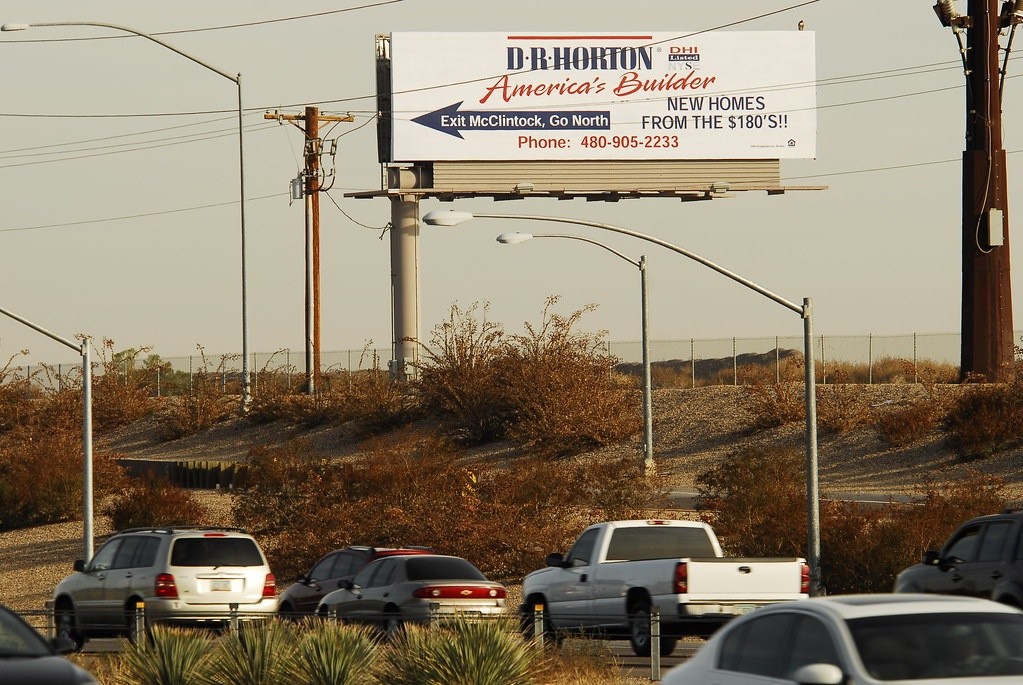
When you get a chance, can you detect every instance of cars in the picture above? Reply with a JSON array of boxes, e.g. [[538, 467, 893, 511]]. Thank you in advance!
[[0, 601, 105, 685], [893, 506, 1023, 612], [314, 551, 508, 631], [279, 542, 434, 624], [52, 524, 282, 650], [659, 590, 1023, 685]]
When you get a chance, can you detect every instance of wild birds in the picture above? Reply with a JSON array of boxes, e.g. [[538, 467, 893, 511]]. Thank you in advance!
[[798, 19, 805, 30]]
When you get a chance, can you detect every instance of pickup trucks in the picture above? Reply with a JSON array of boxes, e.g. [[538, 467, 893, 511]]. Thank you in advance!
[[517, 515, 808, 657]]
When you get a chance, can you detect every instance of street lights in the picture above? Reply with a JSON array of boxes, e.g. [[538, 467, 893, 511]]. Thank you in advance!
[[0, 20, 257, 413], [421, 206, 822, 594], [494, 229, 655, 474]]
